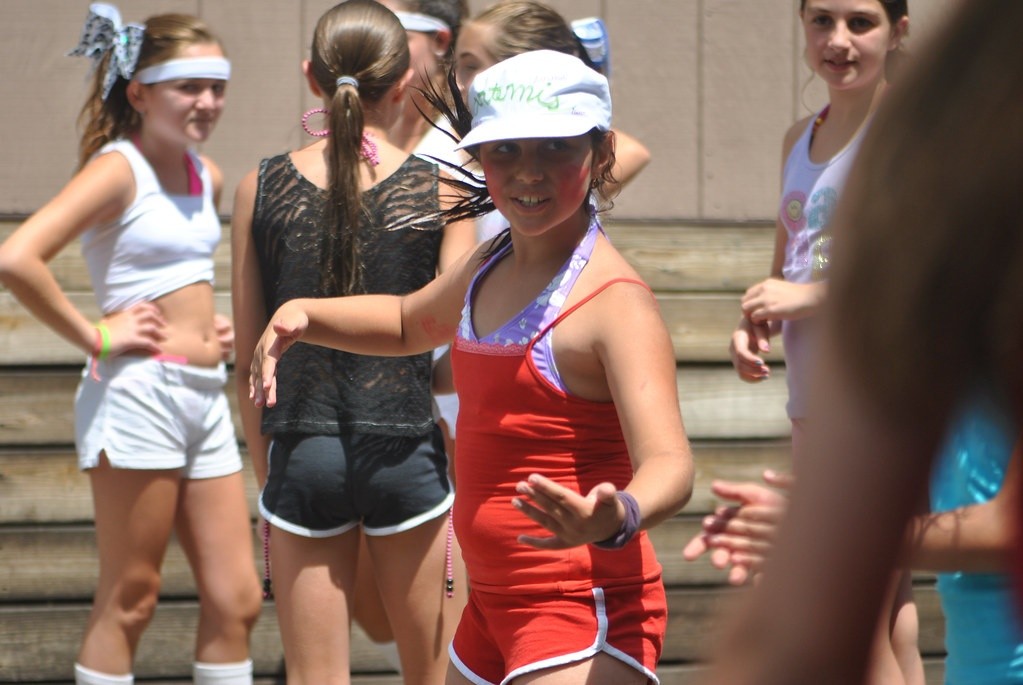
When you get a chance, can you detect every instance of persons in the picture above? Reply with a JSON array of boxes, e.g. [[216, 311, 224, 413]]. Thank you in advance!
[[1, 1, 264, 685], [231, 0, 652, 684], [248, 51, 693, 685], [683, 0, 1023, 684]]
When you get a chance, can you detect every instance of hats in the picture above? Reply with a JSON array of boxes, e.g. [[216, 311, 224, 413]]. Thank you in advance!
[[453, 49, 613, 151]]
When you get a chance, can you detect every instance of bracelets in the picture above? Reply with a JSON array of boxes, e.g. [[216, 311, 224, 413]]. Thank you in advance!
[[88, 321, 111, 385], [592, 491, 641, 552]]
